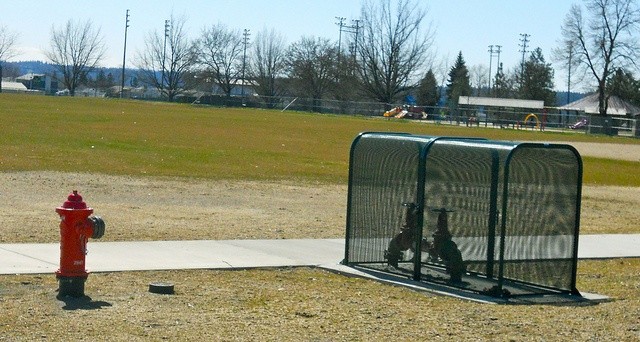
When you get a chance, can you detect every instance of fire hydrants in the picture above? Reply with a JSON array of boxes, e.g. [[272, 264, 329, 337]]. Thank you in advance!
[[55, 190, 105, 302]]
